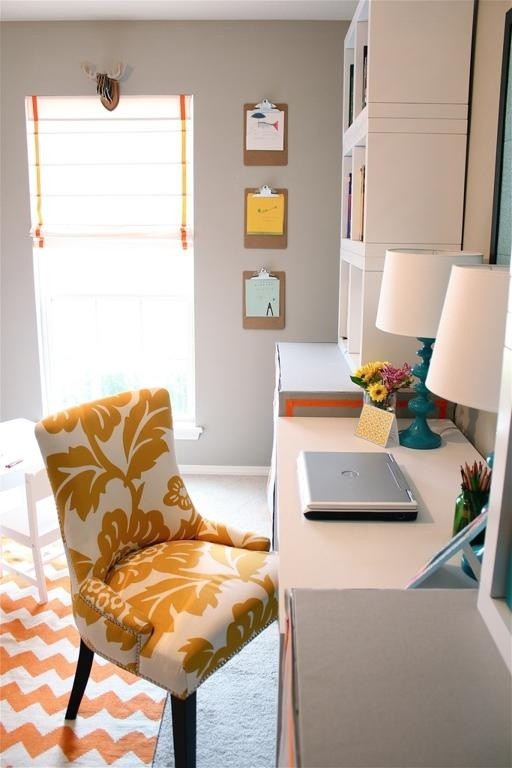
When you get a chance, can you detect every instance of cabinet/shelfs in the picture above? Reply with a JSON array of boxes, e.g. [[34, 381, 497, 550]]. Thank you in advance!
[[272, 0, 478, 418]]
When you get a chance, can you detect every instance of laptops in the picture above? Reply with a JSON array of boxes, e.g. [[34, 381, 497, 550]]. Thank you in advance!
[[297, 451, 419, 521]]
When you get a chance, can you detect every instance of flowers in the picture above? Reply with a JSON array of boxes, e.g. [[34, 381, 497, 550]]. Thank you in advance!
[[351, 357, 416, 409]]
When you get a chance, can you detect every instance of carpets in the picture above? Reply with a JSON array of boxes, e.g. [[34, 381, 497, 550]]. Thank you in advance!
[[1, 535, 172, 768]]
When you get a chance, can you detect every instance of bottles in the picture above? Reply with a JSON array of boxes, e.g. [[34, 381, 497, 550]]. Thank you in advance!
[[453, 485, 487, 544]]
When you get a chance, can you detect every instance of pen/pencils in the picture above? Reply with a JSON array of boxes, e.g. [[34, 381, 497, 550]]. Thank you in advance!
[[457, 460, 492, 546]]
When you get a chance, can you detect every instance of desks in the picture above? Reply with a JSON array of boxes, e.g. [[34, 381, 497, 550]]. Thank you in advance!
[[276, 418, 487, 630]]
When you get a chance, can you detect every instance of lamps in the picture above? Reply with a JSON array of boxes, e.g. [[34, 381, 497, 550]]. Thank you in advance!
[[374, 248, 507, 465]]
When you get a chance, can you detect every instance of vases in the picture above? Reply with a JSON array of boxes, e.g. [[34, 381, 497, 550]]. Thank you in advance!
[[361, 389, 397, 414]]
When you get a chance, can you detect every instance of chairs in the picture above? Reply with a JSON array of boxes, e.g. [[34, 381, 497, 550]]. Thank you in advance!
[[2, 413, 63, 613], [37, 389, 279, 766]]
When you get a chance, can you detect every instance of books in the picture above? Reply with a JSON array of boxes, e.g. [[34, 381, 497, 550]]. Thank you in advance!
[[346, 44, 368, 242]]
